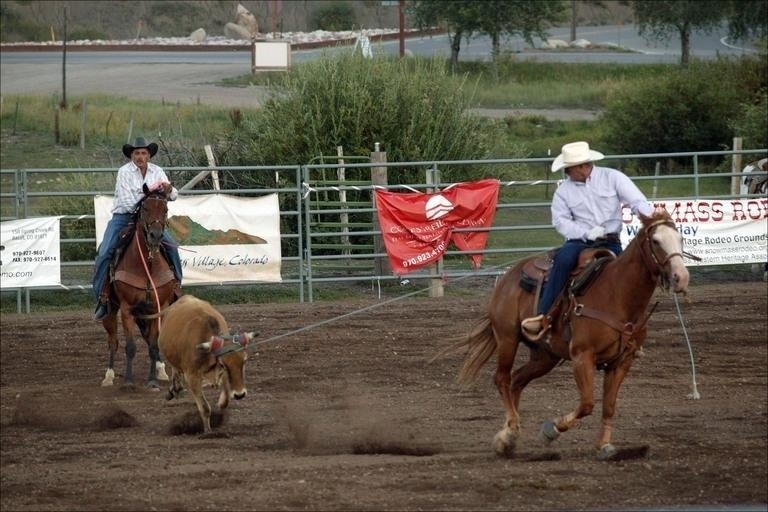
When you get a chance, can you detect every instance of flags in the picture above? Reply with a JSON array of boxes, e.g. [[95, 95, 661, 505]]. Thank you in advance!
[[0, 214, 64, 293], [372, 177, 504, 277]]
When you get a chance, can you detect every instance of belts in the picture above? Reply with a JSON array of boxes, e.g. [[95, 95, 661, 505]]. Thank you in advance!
[[569, 235, 621, 244]]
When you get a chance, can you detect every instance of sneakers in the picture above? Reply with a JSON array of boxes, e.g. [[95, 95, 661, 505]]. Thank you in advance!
[[95, 304, 107, 320], [522, 314, 546, 333]]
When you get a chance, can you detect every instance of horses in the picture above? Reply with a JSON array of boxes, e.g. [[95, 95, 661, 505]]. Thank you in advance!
[[101, 181, 178, 392], [426, 208, 690, 461]]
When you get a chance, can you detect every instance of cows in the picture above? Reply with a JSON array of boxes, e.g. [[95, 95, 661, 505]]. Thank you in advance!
[[139, 294, 260, 434]]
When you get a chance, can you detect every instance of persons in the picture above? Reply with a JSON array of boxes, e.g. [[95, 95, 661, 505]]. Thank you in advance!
[[91, 136, 184, 322], [523, 139, 654, 336]]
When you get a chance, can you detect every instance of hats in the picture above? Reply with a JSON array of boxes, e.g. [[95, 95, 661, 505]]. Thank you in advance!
[[122, 136, 159, 160], [550, 140, 606, 174]]
